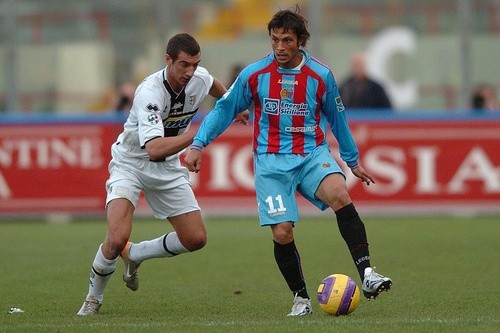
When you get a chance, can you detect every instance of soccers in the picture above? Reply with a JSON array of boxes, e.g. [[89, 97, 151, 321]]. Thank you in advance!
[[147, 114, 160, 125], [316, 274, 361, 316]]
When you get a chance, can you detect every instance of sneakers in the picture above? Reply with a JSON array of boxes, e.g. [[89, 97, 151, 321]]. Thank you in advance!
[[120, 241, 142, 291], [362, 267, 392, 301], [288, 296, 313, 316], [77, 296, 102, 316]]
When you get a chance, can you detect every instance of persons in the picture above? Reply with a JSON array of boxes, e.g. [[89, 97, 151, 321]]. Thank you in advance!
[[184, 10, 393, 317], [77, 34, 250, 316], [111, 84, 134, 113], [338, 55, 390, 109]]
